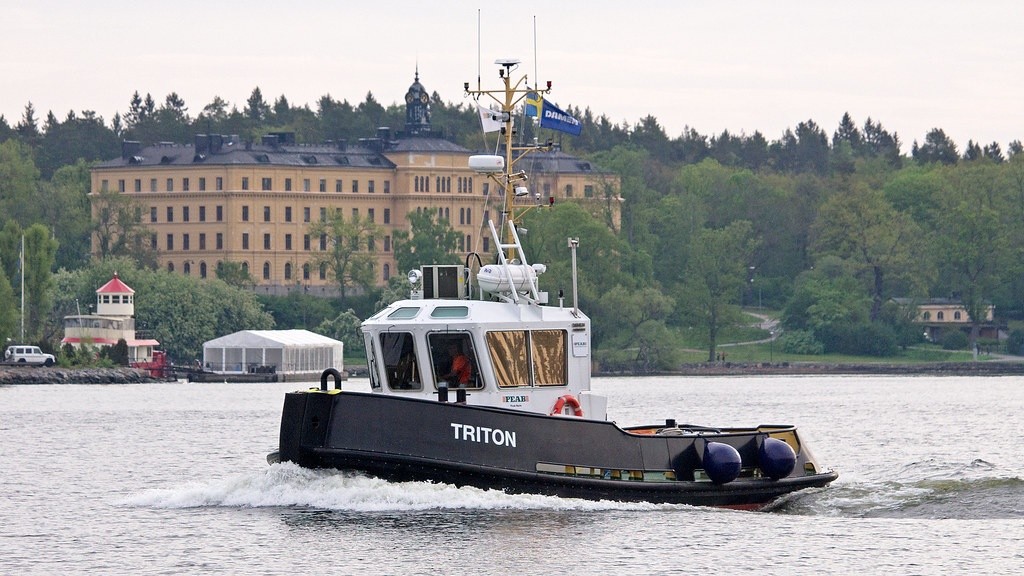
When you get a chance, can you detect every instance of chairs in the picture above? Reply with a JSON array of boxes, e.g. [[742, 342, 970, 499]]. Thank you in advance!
[[258, 366, 276, 373]]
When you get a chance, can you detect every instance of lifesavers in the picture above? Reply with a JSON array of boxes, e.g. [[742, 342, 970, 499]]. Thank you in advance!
[[553, 394, 582, 417], [193, 359, 201, 368]]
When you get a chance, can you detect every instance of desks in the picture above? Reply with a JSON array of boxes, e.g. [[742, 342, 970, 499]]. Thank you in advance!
[[252, 367, 272, 373]]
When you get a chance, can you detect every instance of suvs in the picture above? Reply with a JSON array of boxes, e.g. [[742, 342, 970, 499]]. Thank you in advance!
[[5, 346, 55, 367]]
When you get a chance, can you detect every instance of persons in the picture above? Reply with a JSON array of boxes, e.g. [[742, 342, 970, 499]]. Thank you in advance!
[[716, 352, 726, 362], [977, 343, 990, 355], [442, 342, 472, 388]]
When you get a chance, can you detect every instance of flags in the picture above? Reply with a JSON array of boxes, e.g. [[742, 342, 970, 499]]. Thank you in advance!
[[523, 87, 541, 127], [541, 98, 583, 136], [479, 105, 515, 132]]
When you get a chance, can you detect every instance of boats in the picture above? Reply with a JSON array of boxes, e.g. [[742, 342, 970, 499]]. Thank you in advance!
[[266, 8, 839, 513]]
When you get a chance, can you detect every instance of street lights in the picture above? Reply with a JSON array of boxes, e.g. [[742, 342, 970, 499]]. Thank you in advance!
[[769, 330, 773, 364], [924, 332, 927, 362]]
